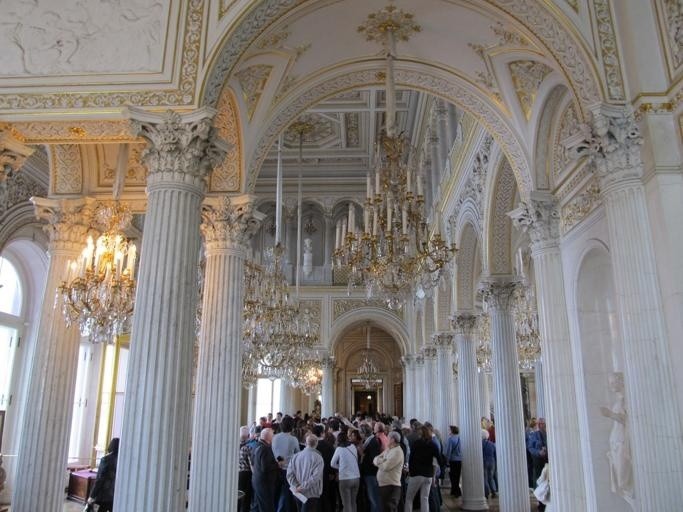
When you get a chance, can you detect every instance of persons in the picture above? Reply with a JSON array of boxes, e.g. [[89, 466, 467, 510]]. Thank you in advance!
[[481, 425, 497, 498], [525, 417, 550, 509], [599, 371, 641, 512], [238, 411, 463, 511], [88, 438, 119, 512]]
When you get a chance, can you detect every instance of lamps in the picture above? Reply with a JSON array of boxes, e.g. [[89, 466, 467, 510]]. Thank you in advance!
[[52, 142, 138, 348], [191, 218, 327, 399], [329, 4, 460, 312], [446, 267, 545, 382], [355, 322, 382, 392]]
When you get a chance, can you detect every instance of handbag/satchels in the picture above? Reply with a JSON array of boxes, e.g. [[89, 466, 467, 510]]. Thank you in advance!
[[439, 454, 447, 469], [84, 502, 94, 512], [533, 480, 550, 505]]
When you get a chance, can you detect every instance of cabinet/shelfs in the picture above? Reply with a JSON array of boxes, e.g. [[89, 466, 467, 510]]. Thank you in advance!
[[67, 468, 97, 507]]
